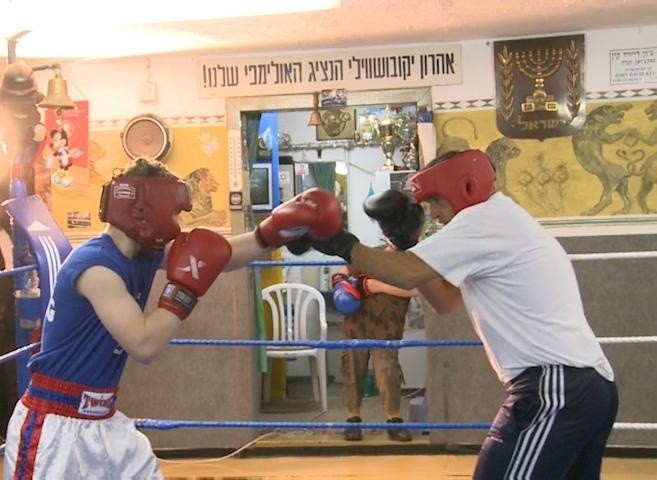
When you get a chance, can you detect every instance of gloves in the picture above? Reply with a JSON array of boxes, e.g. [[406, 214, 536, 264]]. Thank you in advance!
[[286, 228, 359, 263], [158, 228, 232, 321], [332, 273, 362, 315], [255, 188, 342, 250], [363, 190, 424, 249], [349, 275, 371, 298]]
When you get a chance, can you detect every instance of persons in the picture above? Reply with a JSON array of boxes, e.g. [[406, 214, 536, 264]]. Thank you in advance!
[[4, 159, 345, 480], [332, 203, 424, 440], [288, 148, 619, 479]]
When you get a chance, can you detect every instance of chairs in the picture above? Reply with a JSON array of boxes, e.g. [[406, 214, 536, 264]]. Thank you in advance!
[[260, 283, 328, 411]]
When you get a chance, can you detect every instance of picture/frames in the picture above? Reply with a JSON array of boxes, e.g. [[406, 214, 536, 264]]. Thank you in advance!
[[121, 116, 170, 162]]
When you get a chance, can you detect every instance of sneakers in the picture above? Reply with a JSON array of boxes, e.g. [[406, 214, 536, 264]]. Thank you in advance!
[[344, 416, 361, 441], [386, 417, 412, 441]]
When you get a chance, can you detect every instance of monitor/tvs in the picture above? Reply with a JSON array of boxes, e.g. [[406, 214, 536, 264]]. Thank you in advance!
[[250, 162, 273, 211]]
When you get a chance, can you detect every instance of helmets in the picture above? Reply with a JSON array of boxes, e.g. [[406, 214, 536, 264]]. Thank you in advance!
[[407, 150, 495, 216], [100, 173, 192, 246]]
